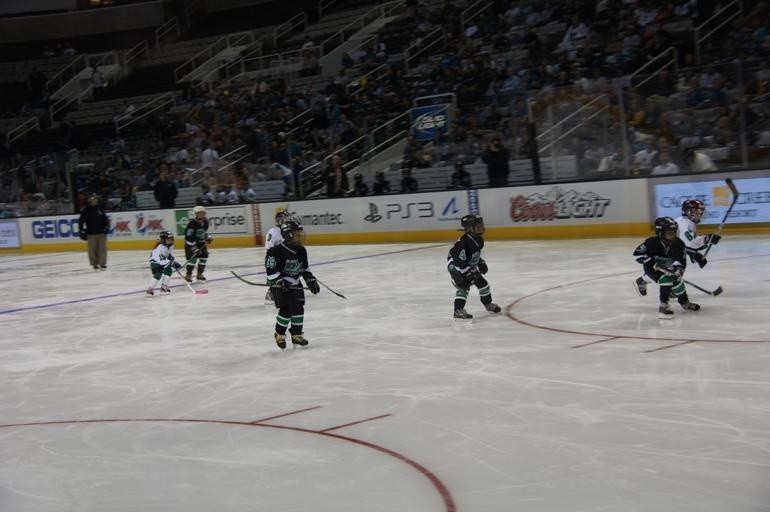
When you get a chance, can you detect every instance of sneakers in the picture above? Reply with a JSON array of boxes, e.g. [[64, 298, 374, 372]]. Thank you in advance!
[[275, 333, 308, 348], [487, 303, 501, 313], [636, 277, 646, 295], [147, 285, 170, 294], [185, 274, 205, 281], [660, 301, 699, 314], [454, 309, 472, 318]]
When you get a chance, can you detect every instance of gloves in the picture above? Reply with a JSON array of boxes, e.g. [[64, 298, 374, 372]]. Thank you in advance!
[[478, 259, 488, 274], [277, 278, 288, 292], [696, 253, 707, 268], [307, 277, 320, 294], [707, 233, 721, 243]]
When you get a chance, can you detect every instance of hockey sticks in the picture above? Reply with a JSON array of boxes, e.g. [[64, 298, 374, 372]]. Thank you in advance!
[[315, 276, 347, 299], [656, 264, 724, 296], [230, 271, 311, 291], [173, 241, 208, 272], [175, 266, 208, 294], [701, 178, 739, 260]]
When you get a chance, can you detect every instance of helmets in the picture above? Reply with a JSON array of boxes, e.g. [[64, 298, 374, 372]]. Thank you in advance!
[[193, 206, 206, 221], [682, 199, 704, 216], [461, 214, 484, 235], [655, 217, 678, 235], [160, 231, 173, 243], [274, 208, 303, 238]]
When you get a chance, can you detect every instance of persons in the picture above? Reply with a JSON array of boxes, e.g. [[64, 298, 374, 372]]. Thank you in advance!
[[146, 231, 181, 296], [78, 196, 110, 268], [631, 216, 700, 316], [635, 200, 720, 294], [265, 207, 290, 301], [184, 206, 213, 282], [0, 0, 769, 210], [447, 214, 501, 320], [266, 215, 320, 349]]
